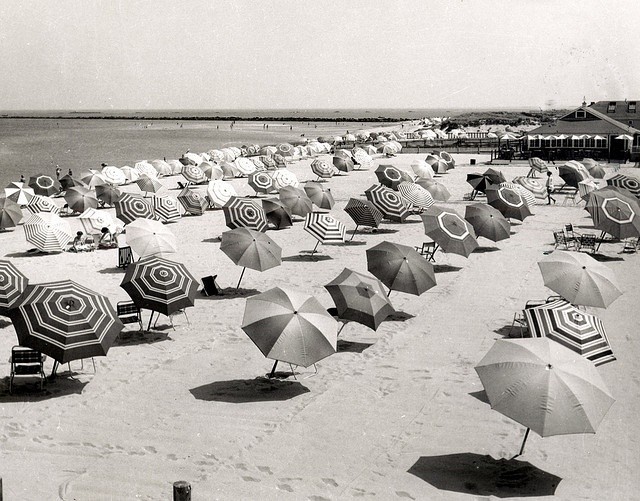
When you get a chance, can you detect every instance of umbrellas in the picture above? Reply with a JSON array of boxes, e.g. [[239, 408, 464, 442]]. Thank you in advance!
[[485, 182, 532, 222], [365, 240, 437, 296], [119, 254, 200, 330], [475, 337, 616, 455], [303, 211, 346, 255], [606, 173, 640, 198], [323, 266, 396, 332], [537, 250, 623, 309], [464, 202, 510, 241], [124, 216, 178, 257], [1, 197, 24, 229], [219, 226, 282, 288], [584, 188, 640, 249], [113, 191, 156, 224], [0, 259, 30, 318], [521, 298, 617, 367], [363, 182, 411, 228], [344, 196, 383, 241], [23, 211, 74, 253], [242, 285, 339, 379], [6, 278, 125, 377], [419, 204, 479, 258], [222, 195, 269, 234], [76, 205, 117, 237]]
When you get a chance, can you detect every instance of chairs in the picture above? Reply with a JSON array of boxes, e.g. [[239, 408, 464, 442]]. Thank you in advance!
[[581, 233, 596, 254], [10, 346, 44, 392], [509, 300, 545, 337], [414, 241, 438, 260], [177, 181, 188, 189], [553, 231, 573, 250], [59, 204, 69, 216], [547, 297, 562, 302], [360, 225, 379, 234], [153, 308, 189, 331], [565, 225, 577, 237], [561, 188, 580, 207], [622, 236, 640, 255], [113, 299, 144, 337]]
